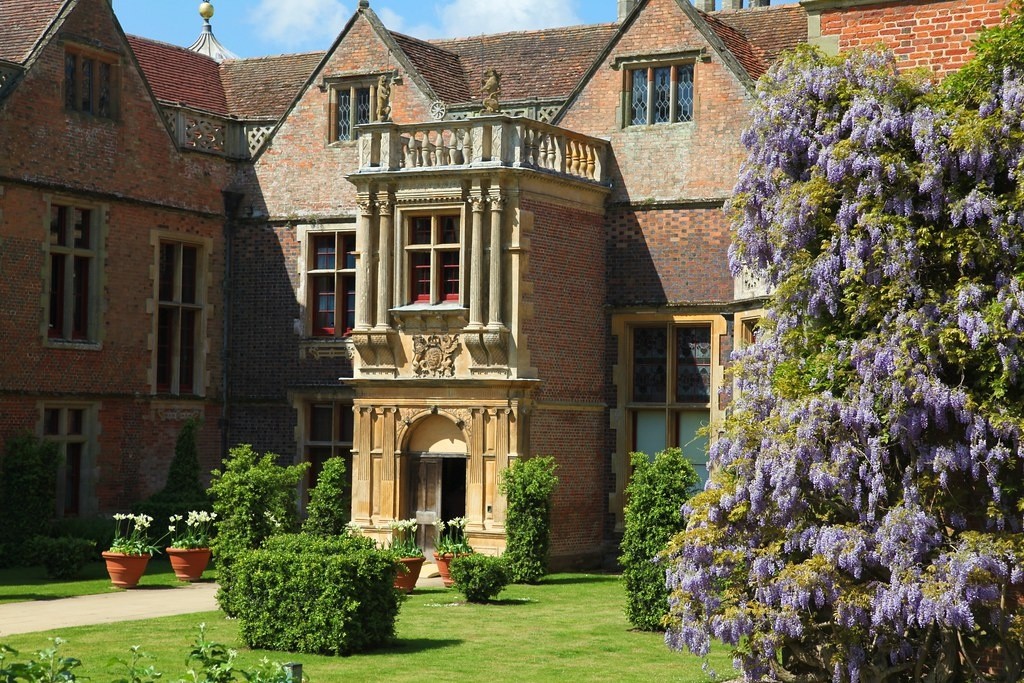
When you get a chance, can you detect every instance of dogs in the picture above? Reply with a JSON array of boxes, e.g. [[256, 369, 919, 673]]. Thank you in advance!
[[478, 67, 502, 114], [376, 74, 392, 123]]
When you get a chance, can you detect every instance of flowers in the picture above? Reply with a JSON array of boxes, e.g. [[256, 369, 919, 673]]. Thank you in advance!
[[376, 516, 426, 559], [111, 509, 217, 558], [431, 516, 473, 560]]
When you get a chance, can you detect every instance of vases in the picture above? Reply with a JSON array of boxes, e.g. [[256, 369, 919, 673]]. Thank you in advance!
[[165, 547, 211, 582], [102, 551, 150, 588], [393, 558, 426, 594], [433, 552, 470, 588]]
